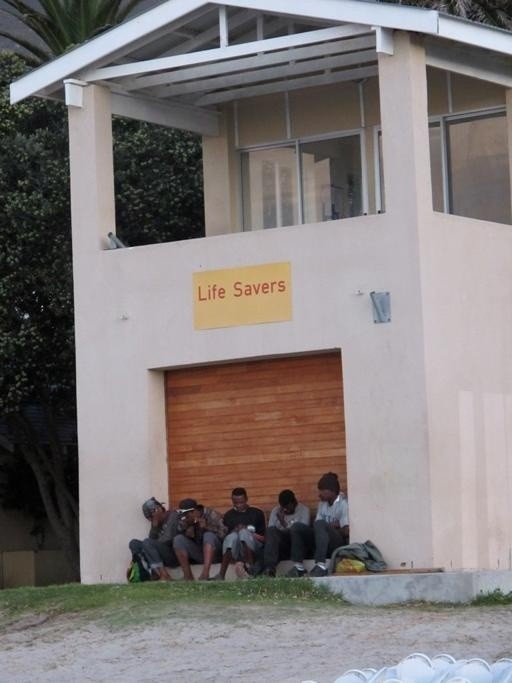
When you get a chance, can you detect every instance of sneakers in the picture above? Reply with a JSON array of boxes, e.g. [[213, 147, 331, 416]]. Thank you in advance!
[[285, 567, 307, 578], [309, 565, 328, 577], [260, 570, 275, 578], [236, 561, 256, 579], [207, 576, 225, 581]]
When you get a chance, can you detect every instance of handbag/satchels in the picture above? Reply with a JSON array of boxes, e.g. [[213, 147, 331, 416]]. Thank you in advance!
[[127, 552, 152, 583]]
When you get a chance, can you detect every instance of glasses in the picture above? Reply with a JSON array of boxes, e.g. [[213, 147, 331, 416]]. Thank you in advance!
[[175, 508, 194, 515]]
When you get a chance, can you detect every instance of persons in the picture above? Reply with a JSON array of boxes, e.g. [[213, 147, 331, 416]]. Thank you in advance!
[[127, 495, 188, 582], [252, 488, 310, 579], [206, 486, 266, 583], [282, 470, 348, 580], [172, 498, 228, 583]]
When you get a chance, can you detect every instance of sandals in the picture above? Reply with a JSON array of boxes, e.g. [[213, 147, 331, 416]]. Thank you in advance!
[[158, 574, 172, 582]]
